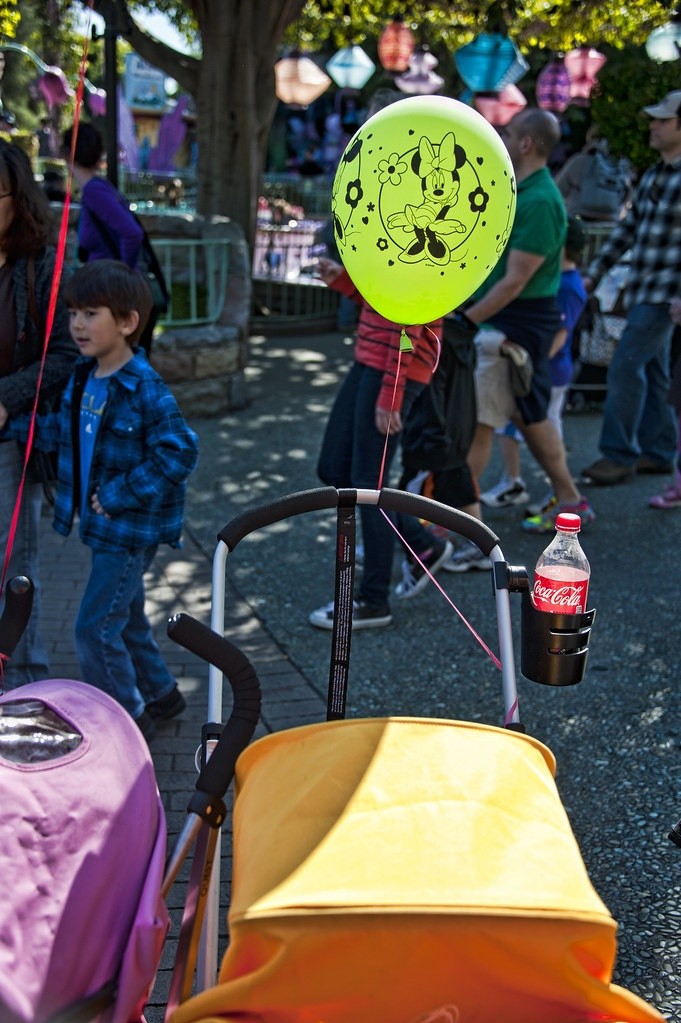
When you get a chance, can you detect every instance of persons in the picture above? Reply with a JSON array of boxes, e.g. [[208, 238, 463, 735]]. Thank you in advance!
[[60, 122, 172, 369], [0, 138, 79, 693], [441, 108, 601, 577], [311, 258, 453, 630], [0, 258, 199, 745], [580, 90, 681, 512]]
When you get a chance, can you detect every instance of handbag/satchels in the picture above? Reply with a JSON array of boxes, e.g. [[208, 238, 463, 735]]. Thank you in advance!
[[15, 369, 60, 484]]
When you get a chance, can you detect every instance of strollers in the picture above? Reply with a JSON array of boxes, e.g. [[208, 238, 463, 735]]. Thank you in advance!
[[165, 483, 666, 1022], [1, 571, 264, 1022], [563, 211, 631, 416]]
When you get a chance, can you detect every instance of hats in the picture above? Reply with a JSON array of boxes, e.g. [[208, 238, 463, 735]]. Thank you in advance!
[[644, 88, 681, 119]]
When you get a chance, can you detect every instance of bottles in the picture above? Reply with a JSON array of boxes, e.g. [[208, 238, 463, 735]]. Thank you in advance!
[[535, 511, 590, 616]]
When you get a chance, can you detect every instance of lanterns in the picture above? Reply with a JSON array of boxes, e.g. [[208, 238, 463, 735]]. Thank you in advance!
[[535, 46, 607, 109], [274, 51, 329, 111], [326, 43, 377, 95], [378, 16, 445, 96], [645, 22, 681, 64], [451, 31, 527, 125]]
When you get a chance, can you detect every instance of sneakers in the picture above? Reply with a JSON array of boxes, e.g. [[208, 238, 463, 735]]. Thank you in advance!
[[396, 538, 453, 601], [633, 450, 677, 476], [523, 498, 598, 533], [310, 592, 394, 630], [441, 537, 492, 573], [524, 482, 561, 517], [478, 475, 530, 507], [581, 455, 633, 487]]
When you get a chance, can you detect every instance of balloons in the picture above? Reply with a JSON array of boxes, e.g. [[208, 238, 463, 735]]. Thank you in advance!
[[329, 94, 517, 328]]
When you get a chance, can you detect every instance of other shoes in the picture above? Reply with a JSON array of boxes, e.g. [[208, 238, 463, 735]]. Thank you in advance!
[[648, 472, 681, 507], [135, 682, 187, 743]]
